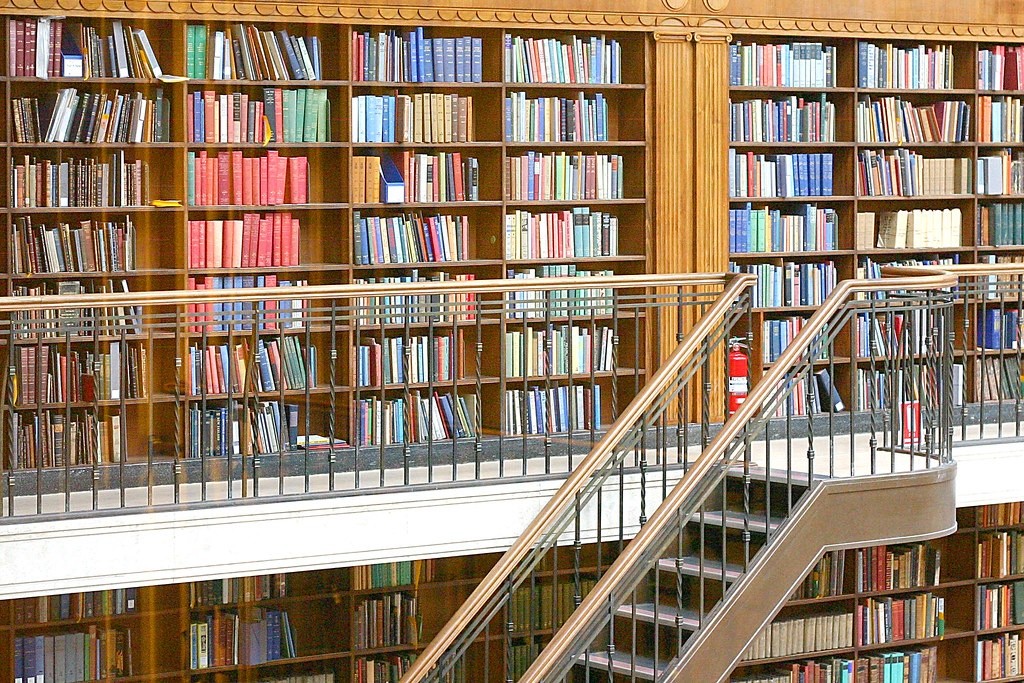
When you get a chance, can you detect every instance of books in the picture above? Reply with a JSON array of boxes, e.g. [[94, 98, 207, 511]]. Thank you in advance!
[[9, 15, 172, 459], [354, 541, 609, 683], [184, 19, 351, 459], [730, 502, 1024, 683], [352, 30, 483, 445], [16, 573, 343, 683], [727, 42, 847, 418], [853, 38, 1024, 417], [501, 31, 625, 441]]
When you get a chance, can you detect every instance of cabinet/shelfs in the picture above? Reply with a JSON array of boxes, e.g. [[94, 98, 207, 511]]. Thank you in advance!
[[0, 0, 1024, 496], [0, 501, 1024, 683]]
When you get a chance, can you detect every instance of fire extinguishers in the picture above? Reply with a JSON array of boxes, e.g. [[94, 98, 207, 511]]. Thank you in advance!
[[727, 335, 753, 418]]
